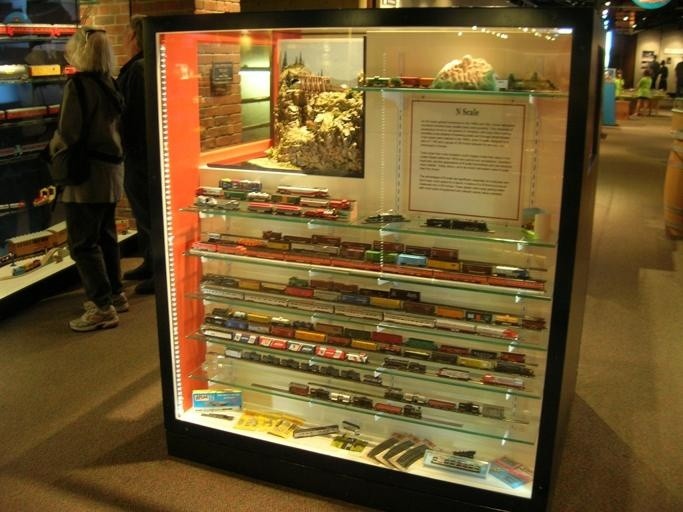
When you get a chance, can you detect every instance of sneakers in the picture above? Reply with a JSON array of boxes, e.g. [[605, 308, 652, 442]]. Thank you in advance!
[[134, 277, 156, 296], [82, 290, 130, 313], [70, 302, 120, 332], [123, 261, 146, 281]]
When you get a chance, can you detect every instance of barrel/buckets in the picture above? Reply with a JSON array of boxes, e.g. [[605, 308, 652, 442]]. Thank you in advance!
[[663, 139, 683, 240]]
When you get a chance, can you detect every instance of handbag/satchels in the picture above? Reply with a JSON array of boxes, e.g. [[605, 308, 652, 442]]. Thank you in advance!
[[44, 142, 74, 186]]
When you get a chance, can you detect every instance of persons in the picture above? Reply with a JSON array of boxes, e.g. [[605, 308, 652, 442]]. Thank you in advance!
[[112, 15, 157, 296], [47, 25, 130, 333], [612, 53, 683, 118]]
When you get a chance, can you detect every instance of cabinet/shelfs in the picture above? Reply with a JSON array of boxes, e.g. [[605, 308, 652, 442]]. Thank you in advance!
[[0, 24, 143, 321], [140, 7, 606, 512]]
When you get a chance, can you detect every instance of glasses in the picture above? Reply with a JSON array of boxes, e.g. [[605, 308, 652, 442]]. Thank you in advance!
[[85, 29, 107, 38]]
